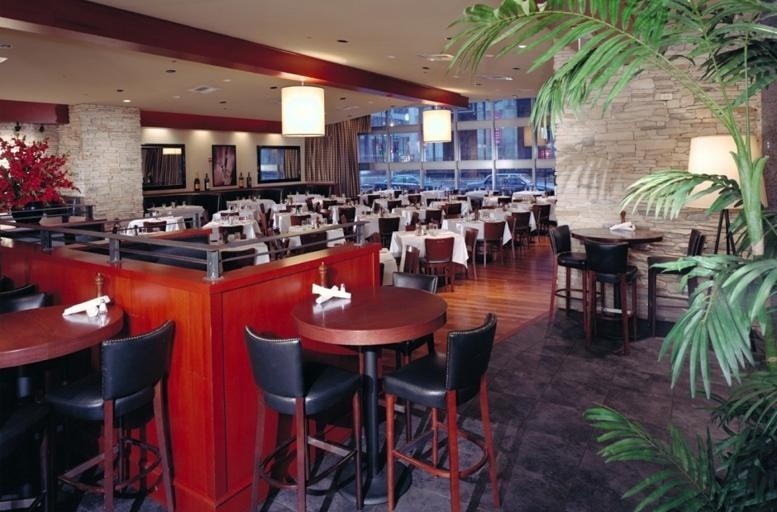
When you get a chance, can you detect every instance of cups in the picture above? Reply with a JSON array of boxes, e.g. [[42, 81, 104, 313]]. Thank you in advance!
[[415, 223, 437, 235]]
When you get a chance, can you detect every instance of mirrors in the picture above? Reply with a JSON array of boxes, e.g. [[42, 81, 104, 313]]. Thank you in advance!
[[141, 143, 187, 192], [257, 145, 301, 184]]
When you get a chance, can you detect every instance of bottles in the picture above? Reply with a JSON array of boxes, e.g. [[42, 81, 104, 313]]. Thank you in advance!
[[247, 172, 251, 187], [239, 172, 244, 188], [204, 172, 210, 191], [194, 171, 200, 192]]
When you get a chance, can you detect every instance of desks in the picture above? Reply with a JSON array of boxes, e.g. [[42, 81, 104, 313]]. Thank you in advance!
[[571, 228, 663, 342], [295, 288, 446, 506], [1, 303, 124, 511], [585, 237, 639, 354]]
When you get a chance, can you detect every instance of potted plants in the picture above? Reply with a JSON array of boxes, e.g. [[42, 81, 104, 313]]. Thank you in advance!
[[0, 134, 82, 222]]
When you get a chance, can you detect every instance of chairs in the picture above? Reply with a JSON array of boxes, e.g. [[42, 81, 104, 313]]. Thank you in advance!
[[550, 225, 594, 336], [31, 321, 178, 512], [242, 326, 369, 512], [383, 312, 499, 512], [647, 228, 705, 340]]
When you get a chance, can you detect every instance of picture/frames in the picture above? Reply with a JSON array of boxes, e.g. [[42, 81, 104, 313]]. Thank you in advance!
[[212, 144, 237, 187]]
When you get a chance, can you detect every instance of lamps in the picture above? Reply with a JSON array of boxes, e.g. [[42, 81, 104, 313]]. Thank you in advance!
[[686, 135, 769, 314], [281, 80, 326, 139], [422, 105, 452, 143]]
